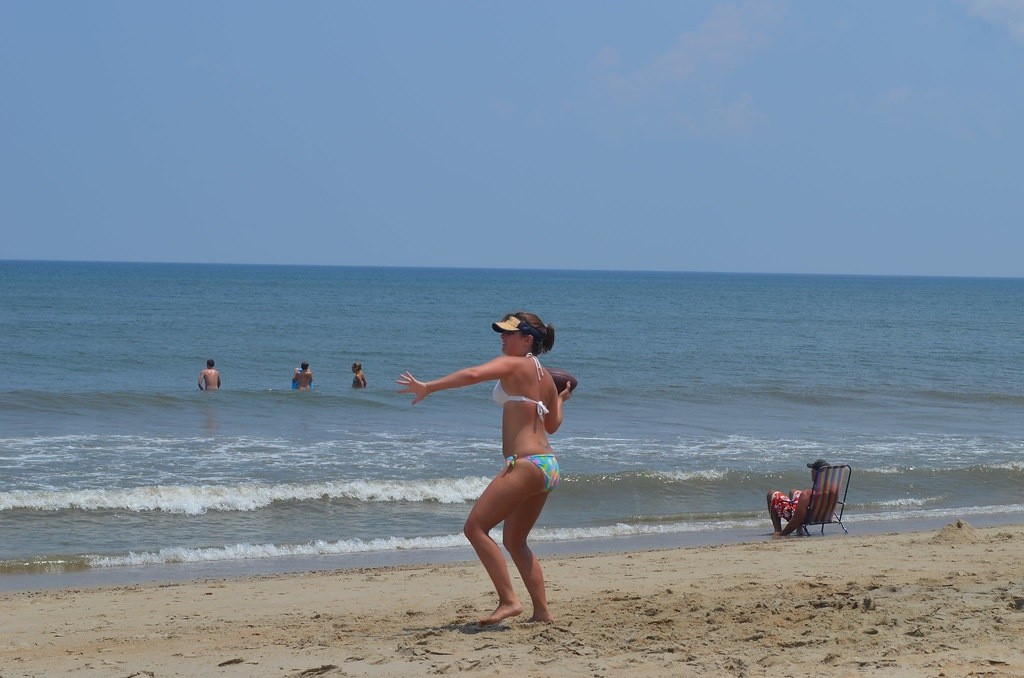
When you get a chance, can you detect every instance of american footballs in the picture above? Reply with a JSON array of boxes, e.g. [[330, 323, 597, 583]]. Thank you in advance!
[[545, 367, 579, 396]]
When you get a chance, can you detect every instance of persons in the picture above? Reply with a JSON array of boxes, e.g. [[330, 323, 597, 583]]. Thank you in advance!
[[293, 362, 313, 391], [198, 359, 221, 390], [352, 362, 367, 388], [767, 459, 830, 536], [396, 312, 574, 627]]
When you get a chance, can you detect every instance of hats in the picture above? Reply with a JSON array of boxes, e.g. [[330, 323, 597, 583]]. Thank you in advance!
[[806, 459, 829, 470], [492, 316, 542, 340]]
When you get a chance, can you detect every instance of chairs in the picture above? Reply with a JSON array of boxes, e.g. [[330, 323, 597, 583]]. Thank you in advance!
[[778, 464, 852, 536]]
